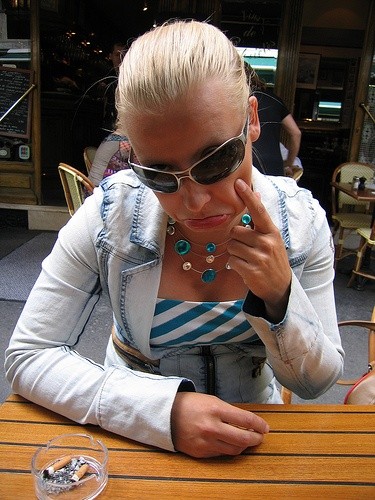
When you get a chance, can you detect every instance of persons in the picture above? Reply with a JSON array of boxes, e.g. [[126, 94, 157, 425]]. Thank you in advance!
[[4, 20, 346, 460], [83, 122, 140, 202], [242, 60, 301, 178], [280, 142, 303, 177]]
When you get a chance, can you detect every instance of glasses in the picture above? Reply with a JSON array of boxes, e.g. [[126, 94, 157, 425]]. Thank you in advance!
[[128, 114, 249, 194]]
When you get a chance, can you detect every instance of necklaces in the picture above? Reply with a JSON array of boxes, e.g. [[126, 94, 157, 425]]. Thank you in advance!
[[165, 214, 255, 283]]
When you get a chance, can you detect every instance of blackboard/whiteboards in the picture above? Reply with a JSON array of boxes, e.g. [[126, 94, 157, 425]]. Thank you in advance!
[[0, 66, 35, 140]]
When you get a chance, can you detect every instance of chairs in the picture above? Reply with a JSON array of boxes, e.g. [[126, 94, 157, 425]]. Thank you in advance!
[[326, 161, 375, 273], [345, 215, 375, 289], [57, 160, 95, 217], [83, 144, 97, 175], [279, 302, 375, 406]]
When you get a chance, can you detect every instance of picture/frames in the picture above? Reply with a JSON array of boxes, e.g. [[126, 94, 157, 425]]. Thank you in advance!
[[296, 52, 320, 90]]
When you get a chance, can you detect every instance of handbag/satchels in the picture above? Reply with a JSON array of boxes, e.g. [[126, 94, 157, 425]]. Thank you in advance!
[[335, 360, 375, 405]]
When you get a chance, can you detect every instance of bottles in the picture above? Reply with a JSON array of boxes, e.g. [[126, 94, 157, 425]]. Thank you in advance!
[[352, 176, 360, 191], [358, 176, 367, 191]]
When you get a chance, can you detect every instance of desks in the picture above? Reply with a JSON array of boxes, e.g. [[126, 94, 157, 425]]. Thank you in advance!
[[0, 393, 375, 500], [330, 181, 375, 284]]
